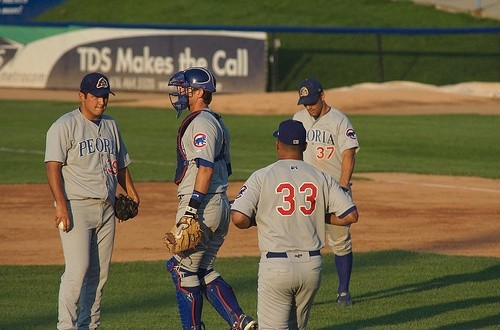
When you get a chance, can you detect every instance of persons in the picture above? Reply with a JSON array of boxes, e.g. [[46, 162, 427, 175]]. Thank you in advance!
[[44, 72, 140, 330], [166, 67, 258, 330], [232, 120, 360, 330], [293, 77, 360, 306]]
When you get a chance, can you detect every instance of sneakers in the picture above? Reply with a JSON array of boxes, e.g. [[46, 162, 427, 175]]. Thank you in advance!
[[231, 313, 258, 330]]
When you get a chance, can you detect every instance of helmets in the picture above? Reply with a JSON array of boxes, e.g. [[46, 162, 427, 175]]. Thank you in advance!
[[167, 67, 218, 92]]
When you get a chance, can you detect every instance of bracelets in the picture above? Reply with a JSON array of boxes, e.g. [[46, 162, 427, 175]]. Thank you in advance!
[[192, 190, 206, 203]]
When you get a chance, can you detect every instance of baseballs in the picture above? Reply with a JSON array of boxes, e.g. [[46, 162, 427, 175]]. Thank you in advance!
[[57, 218, 67, 231]]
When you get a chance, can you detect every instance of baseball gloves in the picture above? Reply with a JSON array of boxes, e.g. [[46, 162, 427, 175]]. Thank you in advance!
[[114, 193, 139, 222], [163, 216, 204, 255]]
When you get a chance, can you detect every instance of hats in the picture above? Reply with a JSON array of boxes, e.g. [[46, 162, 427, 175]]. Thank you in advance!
[[273, 119, 307, 145], [80, 73, 116, 98], [297, 79, 322, 106]]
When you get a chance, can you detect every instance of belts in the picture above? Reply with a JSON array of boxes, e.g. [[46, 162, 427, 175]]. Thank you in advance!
[[266, 250, 321, 258]]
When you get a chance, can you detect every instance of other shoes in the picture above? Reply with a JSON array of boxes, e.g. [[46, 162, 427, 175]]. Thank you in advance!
[[336, 291, 352, 304]]
